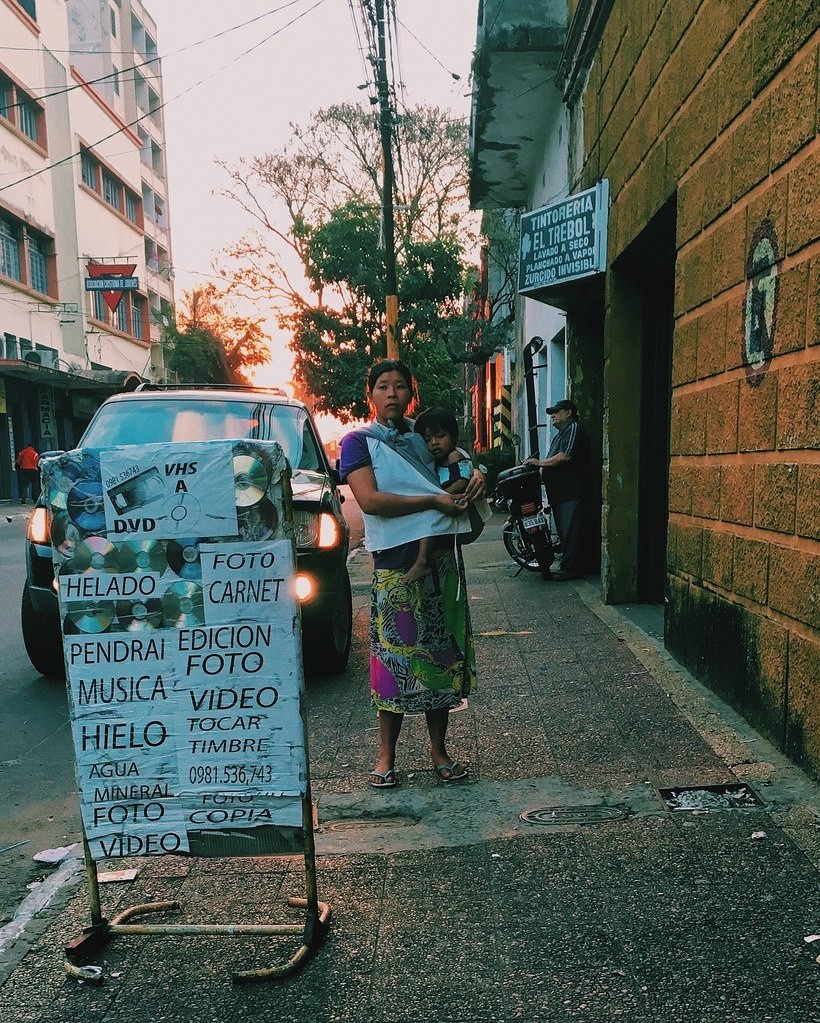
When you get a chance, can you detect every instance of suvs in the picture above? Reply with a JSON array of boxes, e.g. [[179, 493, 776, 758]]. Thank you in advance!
[[21, 383, 364, 681]]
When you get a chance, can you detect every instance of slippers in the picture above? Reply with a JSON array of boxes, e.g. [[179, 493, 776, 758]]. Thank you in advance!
[[371, 771, 396, 787], [436, 761, 469, 780]]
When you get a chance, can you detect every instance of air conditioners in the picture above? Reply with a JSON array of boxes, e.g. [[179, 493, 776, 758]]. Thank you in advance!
[[21, 350, 53, 368]]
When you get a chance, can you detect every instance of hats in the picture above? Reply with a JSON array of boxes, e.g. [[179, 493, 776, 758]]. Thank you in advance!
[[546, 400, 578, 415]]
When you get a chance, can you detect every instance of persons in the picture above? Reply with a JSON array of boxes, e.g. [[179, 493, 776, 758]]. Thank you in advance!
[[337, 357, 479, 788], [521, 400, 594, 583], [17, 441, 41, 505], [397, 407, 493, 585]]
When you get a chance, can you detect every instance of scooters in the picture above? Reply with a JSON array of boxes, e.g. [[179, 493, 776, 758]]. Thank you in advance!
[[488, 445, 560, 586]]
